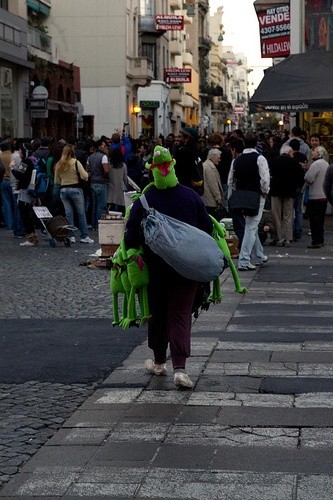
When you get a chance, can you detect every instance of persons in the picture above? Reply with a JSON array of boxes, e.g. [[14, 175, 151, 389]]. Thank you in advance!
[[0, 126, 333, 270], [124, 172, 213, 389]]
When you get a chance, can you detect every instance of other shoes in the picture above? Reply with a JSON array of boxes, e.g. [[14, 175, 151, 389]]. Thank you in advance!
[[307, 244, 322, 248], [322, 243, 325, 246], [285, 241, 290, 247], [269, 240, 279, 244], [19, 241, 39, 246]]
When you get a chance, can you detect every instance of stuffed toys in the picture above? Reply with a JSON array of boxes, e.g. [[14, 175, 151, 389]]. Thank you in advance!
[[111, 204, 152, 329], [201, 214, 248, 311], [130, 146, 178, 199]]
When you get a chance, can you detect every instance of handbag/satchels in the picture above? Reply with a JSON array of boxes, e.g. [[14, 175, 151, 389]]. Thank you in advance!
[[227, 190, 260, 217], [139, 195, 224, 280], [76, 160, 90, 190], [304, 184, 309, 206]]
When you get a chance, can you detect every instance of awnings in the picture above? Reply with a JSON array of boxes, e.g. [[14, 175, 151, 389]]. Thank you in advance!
[[249, 51, 333, 112]]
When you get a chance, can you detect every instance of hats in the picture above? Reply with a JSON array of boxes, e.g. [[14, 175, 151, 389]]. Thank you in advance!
[[179, 127, 197, 138], [23, 142, 34, 151]]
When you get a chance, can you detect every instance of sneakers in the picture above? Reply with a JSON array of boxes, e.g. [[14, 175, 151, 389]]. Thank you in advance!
[[80, 236, 94, 243], [70, 237, 76, 243], [256, 256, 268, 265], [145, 358, 168, 375], [173, 372, 194, 389], [239, 262, 256, 270]]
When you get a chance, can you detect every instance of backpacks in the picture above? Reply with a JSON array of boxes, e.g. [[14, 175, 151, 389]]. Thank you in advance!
[[27, 157, 47, 196]]
